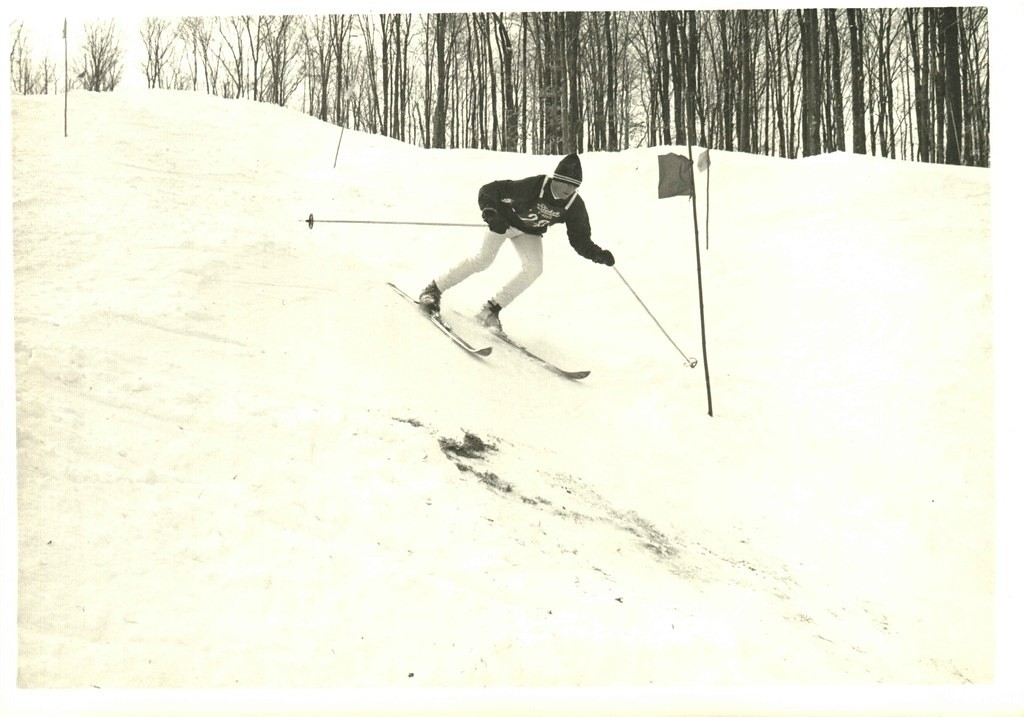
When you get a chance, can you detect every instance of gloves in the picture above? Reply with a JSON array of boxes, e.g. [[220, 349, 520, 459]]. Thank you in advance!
[[590, 245, 615, 267], [482, 208, 510, 234]]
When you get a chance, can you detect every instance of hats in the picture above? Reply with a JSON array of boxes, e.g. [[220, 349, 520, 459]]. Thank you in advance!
[[553, 153, 582, 187]]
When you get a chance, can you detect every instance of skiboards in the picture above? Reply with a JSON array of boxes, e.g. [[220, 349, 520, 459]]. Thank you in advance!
[[387, 282, 591, 381]]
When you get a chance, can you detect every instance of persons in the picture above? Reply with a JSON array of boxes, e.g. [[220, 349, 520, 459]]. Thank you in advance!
[[419, 153, 615, 339]]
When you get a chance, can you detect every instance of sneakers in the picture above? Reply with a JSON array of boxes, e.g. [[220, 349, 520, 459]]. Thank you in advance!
[[477, 299, 502, 331], [419, 280, 442, 318]]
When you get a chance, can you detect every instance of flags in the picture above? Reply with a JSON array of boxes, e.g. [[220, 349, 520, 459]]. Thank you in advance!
[[658, 153, 696, 199], [698, 150, 712, 171]]
